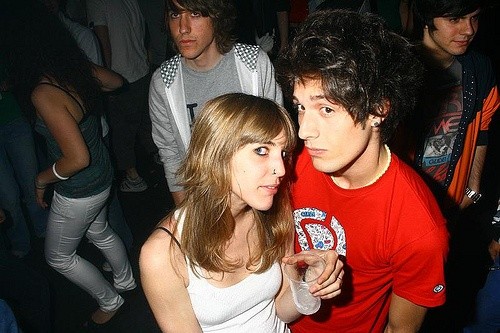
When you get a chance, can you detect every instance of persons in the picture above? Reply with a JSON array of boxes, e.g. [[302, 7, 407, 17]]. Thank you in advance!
[[139, 93, 344, 333], [387, 0, 500, 333], [275, 10, 450, 333], [149, 0, 284, 208], [0, 0, 137, 333], [49, 0, 151, 192]]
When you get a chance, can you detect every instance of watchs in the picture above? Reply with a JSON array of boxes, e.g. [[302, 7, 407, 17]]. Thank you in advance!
[[465, 187, 483, 204]]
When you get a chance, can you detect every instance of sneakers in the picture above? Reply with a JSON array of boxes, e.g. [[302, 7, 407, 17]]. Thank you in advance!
[[119, 179, 147, 192]]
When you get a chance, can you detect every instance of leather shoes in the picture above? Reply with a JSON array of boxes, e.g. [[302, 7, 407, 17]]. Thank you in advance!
[[81, 301, 130, 333]]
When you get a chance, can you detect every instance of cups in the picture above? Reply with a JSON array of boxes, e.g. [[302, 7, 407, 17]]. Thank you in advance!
[[284, 252, 327, 315]]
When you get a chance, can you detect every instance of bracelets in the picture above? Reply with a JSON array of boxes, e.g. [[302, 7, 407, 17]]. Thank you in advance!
[[52, 162, 70, 180], [33, 175, 46, 189]]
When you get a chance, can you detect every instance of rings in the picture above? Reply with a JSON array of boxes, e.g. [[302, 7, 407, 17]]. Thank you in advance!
[[337, 276, 343, 283]]
